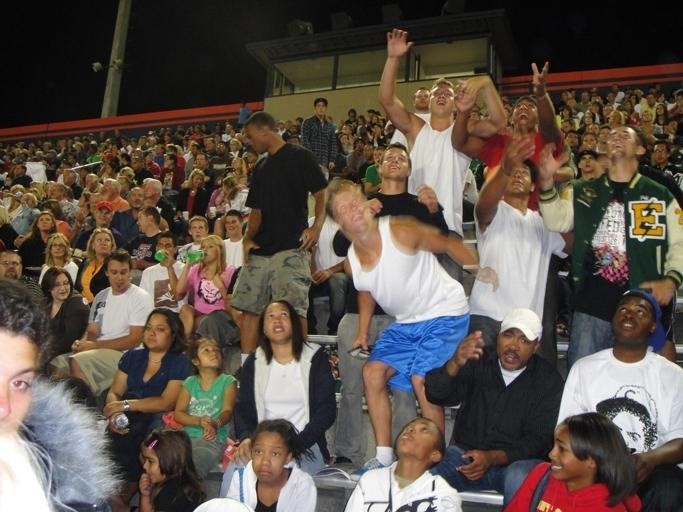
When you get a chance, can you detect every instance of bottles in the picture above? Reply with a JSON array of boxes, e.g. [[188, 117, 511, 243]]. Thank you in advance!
[[186, 250, 207, 261], [153, 249, 166, 261]]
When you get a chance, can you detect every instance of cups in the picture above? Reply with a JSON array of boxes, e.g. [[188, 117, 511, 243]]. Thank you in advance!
[[209, 207, 217, 219], [114, 415, 129, 428], [182, 211, 188, 222]]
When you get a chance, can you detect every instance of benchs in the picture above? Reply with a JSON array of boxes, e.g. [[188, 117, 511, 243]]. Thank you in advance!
[[1, 220, 683, 511]]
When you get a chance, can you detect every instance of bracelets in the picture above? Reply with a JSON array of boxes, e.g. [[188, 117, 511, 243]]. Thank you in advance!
[[534, 92, 547, 100]]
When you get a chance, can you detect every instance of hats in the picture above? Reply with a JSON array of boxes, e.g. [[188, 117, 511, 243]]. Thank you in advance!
[[95, 199, 116, 214], [498, 305, 545, 344], [620, 287, 666, 353], [596, 383, 662, 432]]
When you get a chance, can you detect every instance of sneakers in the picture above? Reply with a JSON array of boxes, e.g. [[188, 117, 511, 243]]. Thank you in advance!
[[348, 456, 388, 481]]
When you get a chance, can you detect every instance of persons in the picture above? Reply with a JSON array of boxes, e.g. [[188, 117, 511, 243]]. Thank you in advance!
[[377, 27, 508, 284], [446, 61, 567, 370]]
